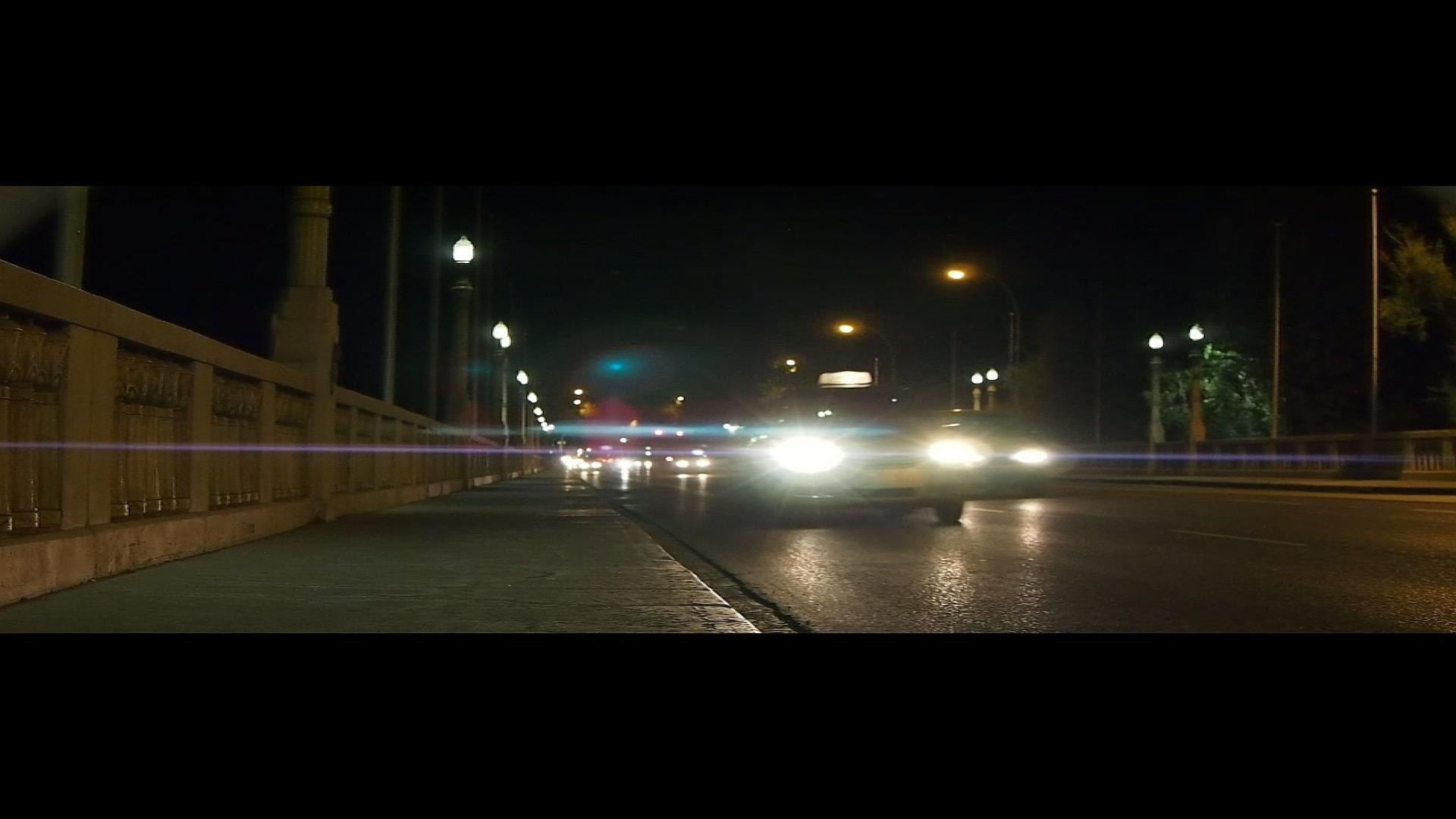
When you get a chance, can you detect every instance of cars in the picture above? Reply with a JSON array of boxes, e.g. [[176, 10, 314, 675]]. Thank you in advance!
[[738, 370, 1050, 529]]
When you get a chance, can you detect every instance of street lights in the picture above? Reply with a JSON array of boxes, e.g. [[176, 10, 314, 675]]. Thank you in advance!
[[946, 267, 1022, 367], [449, 232, 477, 427], [1184, 324, 1210, 444], [836, 322, 898, 384], [1145, 331, 1170, 446], [489, 319, 552, 448]]
